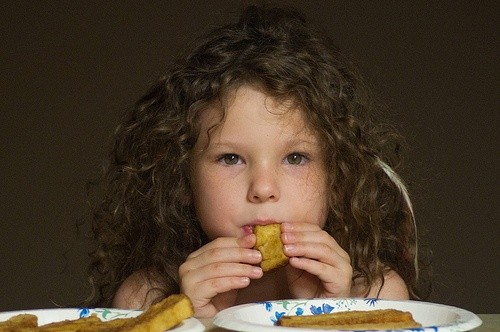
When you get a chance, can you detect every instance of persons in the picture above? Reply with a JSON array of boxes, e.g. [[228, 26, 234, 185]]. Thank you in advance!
[[68, 6, 433, 318]]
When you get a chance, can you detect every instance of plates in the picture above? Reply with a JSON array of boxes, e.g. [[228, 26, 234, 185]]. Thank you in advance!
[[0, 308, 205, 332], [212, 297, 482, 332]]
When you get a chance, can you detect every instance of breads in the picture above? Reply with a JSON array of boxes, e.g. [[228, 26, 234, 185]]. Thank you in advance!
[[0, 293, 196, 332], [252, 224, 291, 272], [275, 309, 423, 331]]
